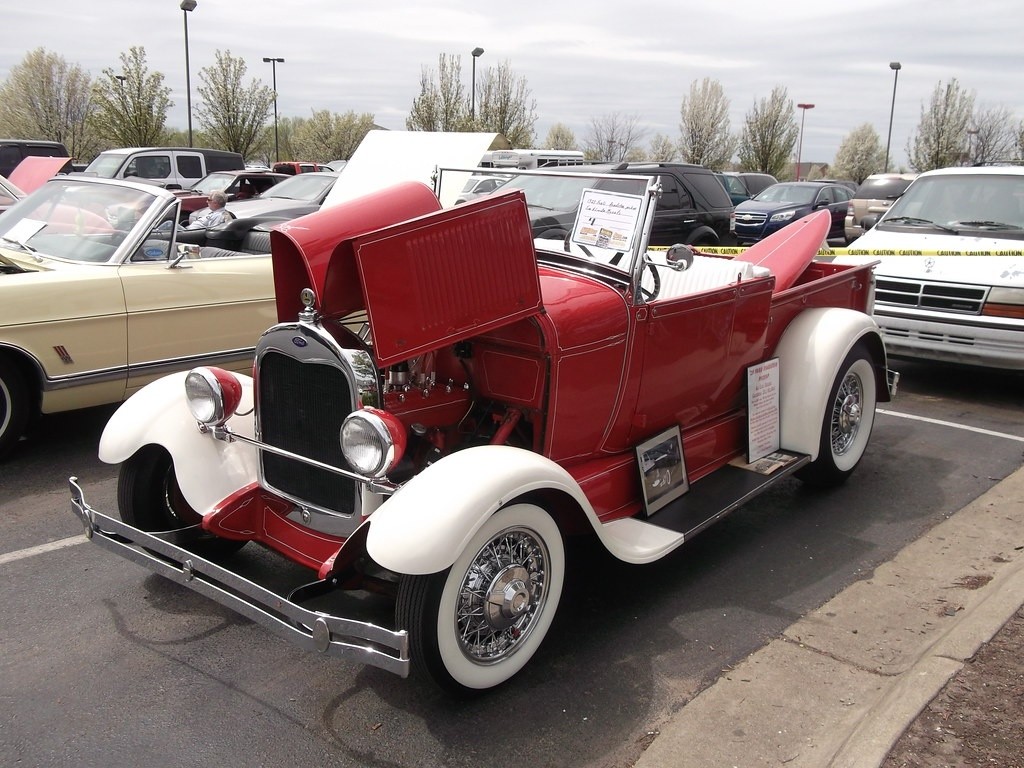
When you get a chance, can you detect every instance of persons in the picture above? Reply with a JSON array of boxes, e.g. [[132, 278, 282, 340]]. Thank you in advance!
[[151, 189, 233, 231]]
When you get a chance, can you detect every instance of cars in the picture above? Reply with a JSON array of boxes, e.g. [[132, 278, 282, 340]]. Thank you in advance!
[[844, 172, 919, 244], [0, 156, 116, 233], [731, 181, 856, 246], [134, 171, 290, 226], [67, 154, 902, 702], [188, 171, 344, 229], [0, 130, 514, 467], [713, 170, 778, 207], [828, 164, 1024, 373], [455, 175, 511, 208]]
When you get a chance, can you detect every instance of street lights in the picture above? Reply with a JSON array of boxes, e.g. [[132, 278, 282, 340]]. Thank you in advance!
[[116, 76, 126, 147], [797, 103, 815, 182], [263, 57, 285, 163], [884, 62, 902, 173], [180, 0, 198, 149], [471, 47, 485, 121]]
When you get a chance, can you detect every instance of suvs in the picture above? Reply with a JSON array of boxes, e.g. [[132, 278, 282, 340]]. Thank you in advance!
[[486, 159, 736, 248]]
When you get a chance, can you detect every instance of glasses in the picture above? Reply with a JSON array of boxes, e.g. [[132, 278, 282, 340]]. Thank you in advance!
[[207, 199, 212, 201]]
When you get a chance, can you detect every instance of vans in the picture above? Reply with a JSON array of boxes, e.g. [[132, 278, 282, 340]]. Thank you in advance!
[[478, 149, 585, 170], [82, 146, 247, 193], [0, 139, 77, 180], [245, 159, 348, 177]]
